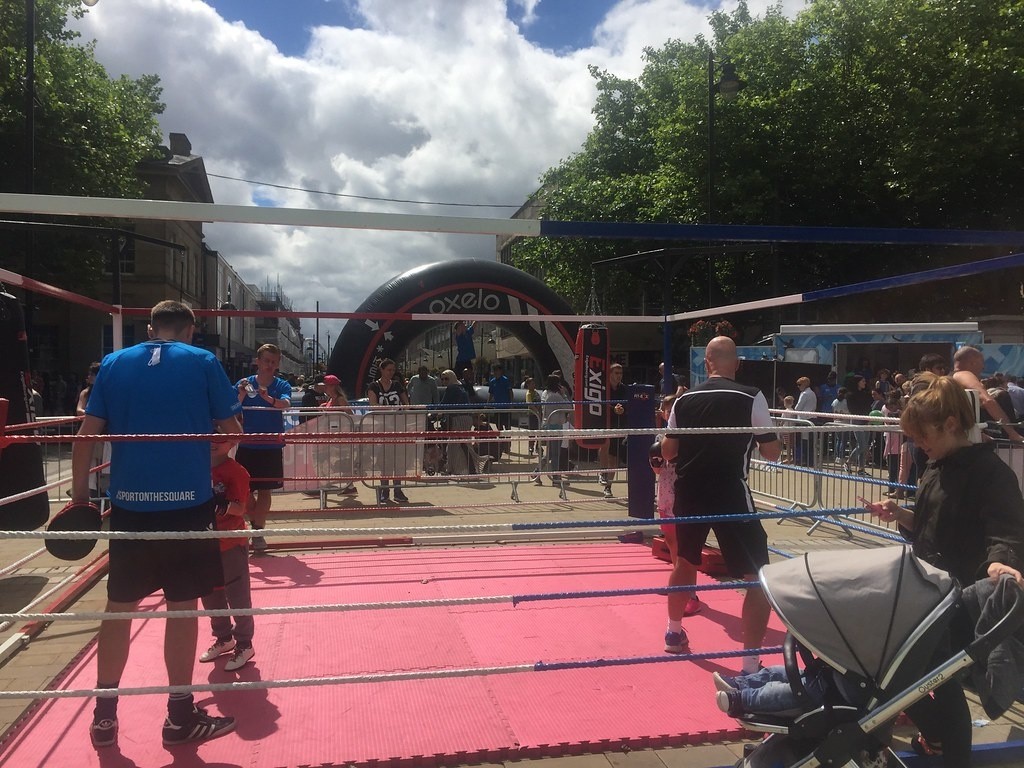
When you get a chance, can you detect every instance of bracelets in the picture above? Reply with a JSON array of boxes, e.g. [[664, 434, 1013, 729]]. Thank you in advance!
[[271, 398, 276, 407]]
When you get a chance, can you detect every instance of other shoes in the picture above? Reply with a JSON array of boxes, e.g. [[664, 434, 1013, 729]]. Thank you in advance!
[[716, 688, 742, 714], [302, 486, 408, 502], [712, 671, 740, 692], [884, 489, 915, 501]]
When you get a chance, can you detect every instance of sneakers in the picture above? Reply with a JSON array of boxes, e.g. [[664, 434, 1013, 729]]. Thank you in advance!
[[665, 625, 690, 653], [739, 661, 769, 677], [551, 480, 570, 486], [683, 595, 702, 617], [843, 462, 851, 477], [251, 533, 267, 549], [162, 705, 237, 745], [199, 635, 238, 662], [856, 469, 873, 478], [598, 472, 608, 485], [529, 471, 542, 484], [603, 488, 613, 496], [89, 719, 118, 746], [223, 640, 255, 671]]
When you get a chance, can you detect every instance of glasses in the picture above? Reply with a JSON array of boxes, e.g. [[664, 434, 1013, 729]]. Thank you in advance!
[[932, 366, 946, 371], [798, 383, 803, 387], [441, 377, 449, 381]]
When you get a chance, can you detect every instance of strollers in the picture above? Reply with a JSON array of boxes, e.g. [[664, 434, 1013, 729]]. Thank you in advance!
[[716, 541, 1024, 768]]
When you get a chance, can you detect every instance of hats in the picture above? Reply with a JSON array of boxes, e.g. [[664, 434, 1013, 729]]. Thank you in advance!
[[323, 375, 340, 384], [309, 374, 325, 384]]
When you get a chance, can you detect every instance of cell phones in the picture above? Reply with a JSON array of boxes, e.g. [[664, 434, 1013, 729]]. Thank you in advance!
[[857, 495, 889, 518]]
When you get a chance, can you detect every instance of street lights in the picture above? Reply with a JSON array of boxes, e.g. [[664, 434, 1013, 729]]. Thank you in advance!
[[220, 292, 237, 369]]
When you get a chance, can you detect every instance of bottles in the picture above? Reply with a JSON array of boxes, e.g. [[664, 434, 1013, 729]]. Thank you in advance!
[[245, 383, 256, 398]]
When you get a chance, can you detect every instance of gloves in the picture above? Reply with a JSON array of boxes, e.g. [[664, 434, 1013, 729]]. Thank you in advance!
[[211, 489, 231, 517]]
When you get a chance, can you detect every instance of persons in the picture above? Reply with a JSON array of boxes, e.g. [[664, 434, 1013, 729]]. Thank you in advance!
[[368, 357, 408, 502], [529, 369, 572, 486], [867, 376, 1024, 768], [817, 347, 1024, 499], [658, 362, 683, 396], [521, 376, 544, 455], [233, 344, 291, 550], [778, 376, 818, 466], [597, 364, 628, 498], [406, 366, 439, 447], [661, 336, 781, 677], [199, 413, 254, 670], [655, 396, 701, 615], [68, 300, 242, 746], [278, 372, 358, 497], [454, 320, 480, 379], [429, 368, 494, 483], [487, 364, 514, 452], [713, 665, 851, 714]]
